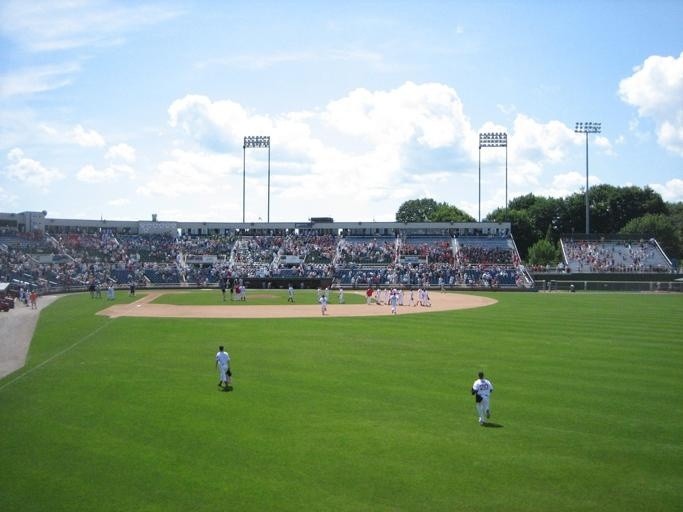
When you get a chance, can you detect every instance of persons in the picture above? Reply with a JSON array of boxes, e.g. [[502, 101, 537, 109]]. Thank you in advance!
[[213, 344, 231, 388], [472, 371, 493, 426]]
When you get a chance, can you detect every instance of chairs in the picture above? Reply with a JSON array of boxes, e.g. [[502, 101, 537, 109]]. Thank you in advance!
[[0, 229, 516, 292], [563, 236, 670, 273]]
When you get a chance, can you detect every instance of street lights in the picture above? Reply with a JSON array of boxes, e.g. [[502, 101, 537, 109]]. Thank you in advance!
[[241, 135, 270, 224], [573, 120, 603, 233], [477, 131, 508, 224]]
